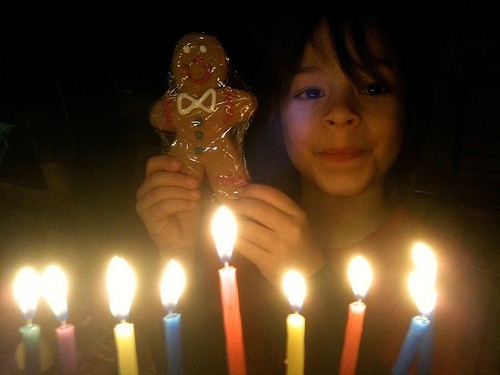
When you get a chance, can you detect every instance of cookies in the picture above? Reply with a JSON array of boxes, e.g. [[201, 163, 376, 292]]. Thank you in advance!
[[149, 33, 258, 201]]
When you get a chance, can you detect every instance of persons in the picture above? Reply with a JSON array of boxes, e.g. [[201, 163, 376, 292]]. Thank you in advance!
[[135, 0, 500, 375]]
